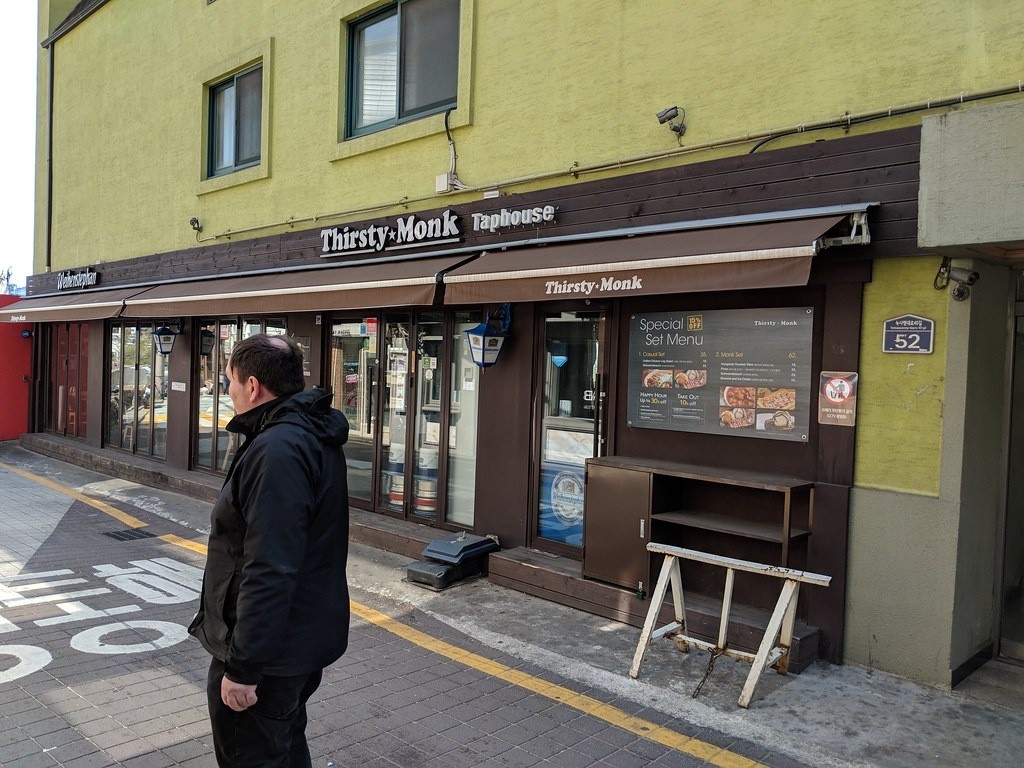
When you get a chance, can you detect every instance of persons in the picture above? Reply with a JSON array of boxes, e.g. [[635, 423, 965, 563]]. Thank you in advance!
[[186, 333, 351, 768], [141, 368, 229, 404]]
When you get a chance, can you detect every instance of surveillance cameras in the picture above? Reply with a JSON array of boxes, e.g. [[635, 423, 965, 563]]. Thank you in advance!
[[190, 217, 197, 225], [948, 267, 979, 285], [656, 106, 678, 124]]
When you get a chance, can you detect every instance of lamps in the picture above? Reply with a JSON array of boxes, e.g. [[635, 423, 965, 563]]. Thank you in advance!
[[462, 303, 511, 374], [189, 217, 202, 231], [201, 325, 216, 359], [150, 317, 185, 358], [656, 105, 686, 137]]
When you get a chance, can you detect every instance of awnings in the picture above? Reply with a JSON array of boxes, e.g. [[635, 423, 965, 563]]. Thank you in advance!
[[1, 283, 159, 324], [117, 251, 477, 318], [445, 211, 854, 306]]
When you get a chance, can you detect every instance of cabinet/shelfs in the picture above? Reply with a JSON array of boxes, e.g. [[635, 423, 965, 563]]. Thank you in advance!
[[581, 455, 814, 600]]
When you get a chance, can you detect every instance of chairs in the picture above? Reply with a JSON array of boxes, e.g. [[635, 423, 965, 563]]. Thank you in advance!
[[222, 422, 245, 472], [124, 404, 158, 452], [67, 386, 87, 435]]
